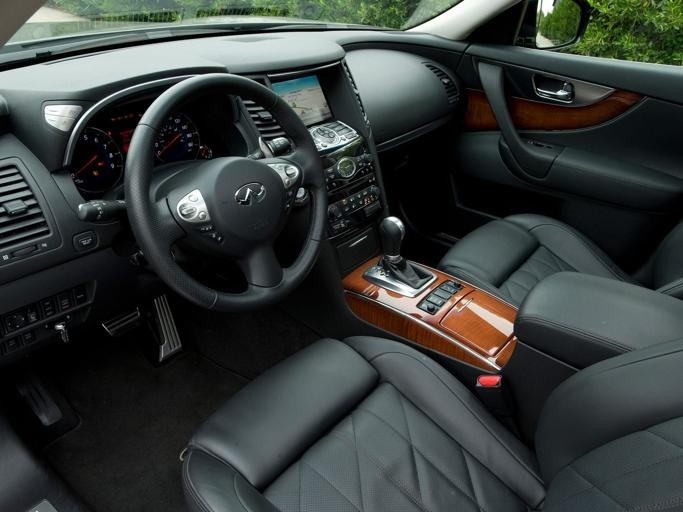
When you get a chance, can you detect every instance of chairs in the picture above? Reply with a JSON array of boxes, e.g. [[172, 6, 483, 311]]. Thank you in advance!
[[179, 333, 683, 511], [442, 214, 683, 308]]
